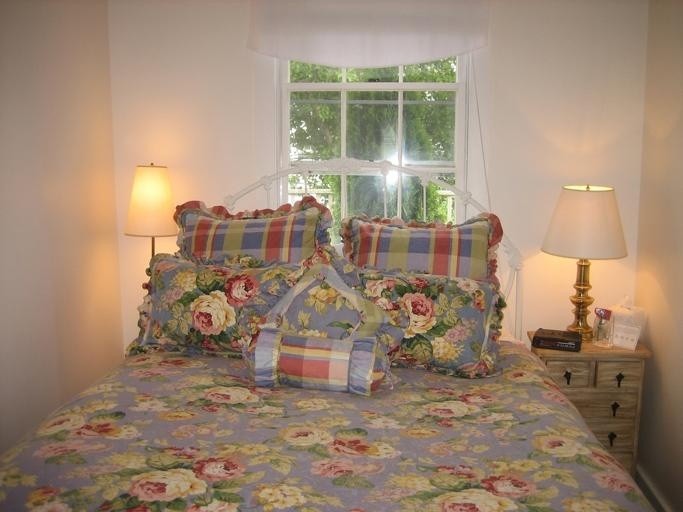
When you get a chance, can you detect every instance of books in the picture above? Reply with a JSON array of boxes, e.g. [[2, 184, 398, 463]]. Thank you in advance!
[[530, 325, 583, 354]]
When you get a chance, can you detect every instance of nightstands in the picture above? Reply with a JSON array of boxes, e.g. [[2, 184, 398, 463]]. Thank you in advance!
[[527, 330, 652, 479]]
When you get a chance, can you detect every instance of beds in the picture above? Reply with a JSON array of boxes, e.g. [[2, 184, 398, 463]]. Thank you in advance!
[[0, 159, 656, 512]]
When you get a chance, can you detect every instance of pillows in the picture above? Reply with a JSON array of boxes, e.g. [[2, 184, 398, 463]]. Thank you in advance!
[[341, 212, 504, 290], [131, 252, 304, 358], [259, 258, 383, 341], [245, 330, 387, 398], [173, 195, 334, 262], [332, 259, 498, 380]]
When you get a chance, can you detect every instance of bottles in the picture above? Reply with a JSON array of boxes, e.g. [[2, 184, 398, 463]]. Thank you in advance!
[[592, 309, 614, 348]]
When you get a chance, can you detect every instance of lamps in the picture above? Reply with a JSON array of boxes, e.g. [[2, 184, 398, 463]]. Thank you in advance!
[[541, 183, 627, 342], [124, 163, 179, 275]]
